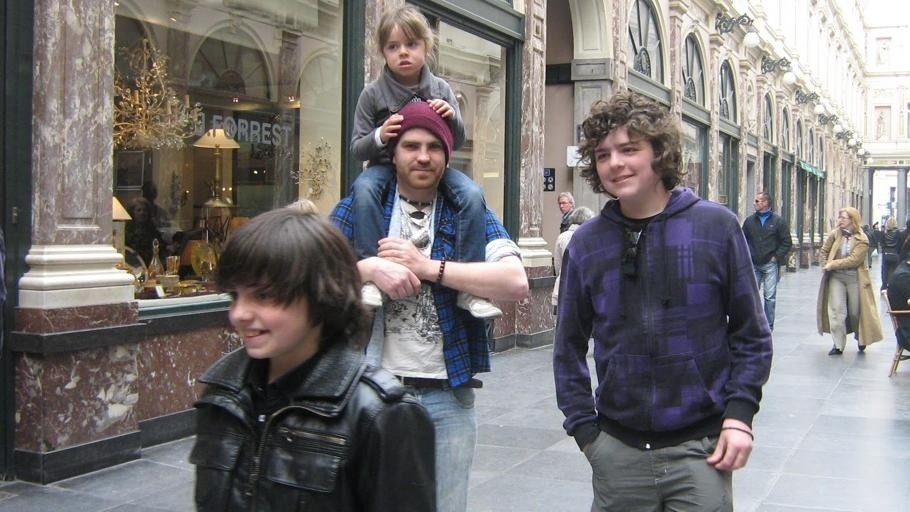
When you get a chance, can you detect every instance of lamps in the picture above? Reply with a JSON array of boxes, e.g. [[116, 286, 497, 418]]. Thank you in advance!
[[112, 196, 132, 221], [192, 129, 240, 199]]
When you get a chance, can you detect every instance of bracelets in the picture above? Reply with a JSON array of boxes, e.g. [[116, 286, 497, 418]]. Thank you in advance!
[[721, 425, 754, 442], [435, 256, 447, 290]]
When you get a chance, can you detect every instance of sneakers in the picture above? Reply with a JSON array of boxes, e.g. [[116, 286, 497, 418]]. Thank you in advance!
[[829, 347, 842, 355], [859, 346, 866, 350], [457, 288, 504, 319], [360, 283, 384, 307]]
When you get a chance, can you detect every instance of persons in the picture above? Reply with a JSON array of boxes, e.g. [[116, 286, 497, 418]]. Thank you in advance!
[[125, 181, 236, 281], [188, 197, 437, 511], [347, 5, 505, 319], [328, 100, 530, 511], [550, 205, 595, 339], [550, 88, 773, 512], [553, 191, 578, 277], [741, 191, 792, 333], [816, 207, 909, 357]]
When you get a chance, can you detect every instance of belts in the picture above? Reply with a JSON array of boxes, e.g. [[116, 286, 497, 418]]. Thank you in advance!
[[394, 374, 484, 391]]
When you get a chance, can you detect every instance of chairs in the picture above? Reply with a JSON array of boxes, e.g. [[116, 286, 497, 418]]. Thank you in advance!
[[881, 290, 910, 377]]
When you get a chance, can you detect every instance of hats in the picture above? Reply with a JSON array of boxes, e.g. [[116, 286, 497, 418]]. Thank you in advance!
[[392, 102, 453, 167]]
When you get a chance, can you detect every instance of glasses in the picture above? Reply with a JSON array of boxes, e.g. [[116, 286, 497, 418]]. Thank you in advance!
[[838, 217, 850, 220], [558, 202, 571, 206], [755, 200, 765, 203], [622, 221, 646, 266]]
